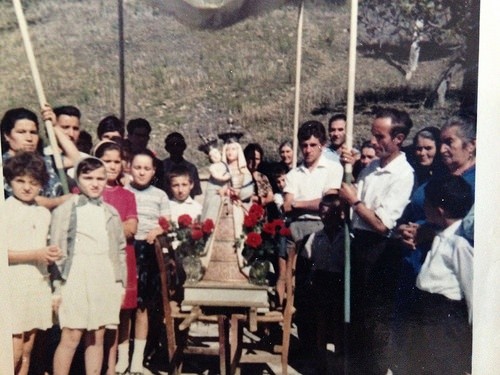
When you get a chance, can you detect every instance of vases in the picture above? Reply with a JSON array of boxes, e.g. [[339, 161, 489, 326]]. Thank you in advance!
[[248, 259, 273, 285], [184, 253, 200, 282]]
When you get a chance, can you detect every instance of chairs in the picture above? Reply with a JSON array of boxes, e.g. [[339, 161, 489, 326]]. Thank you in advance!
[[154, 231, 294, 375]]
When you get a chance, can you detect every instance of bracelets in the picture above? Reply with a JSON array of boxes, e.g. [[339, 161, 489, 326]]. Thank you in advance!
[[352, 201, 362, 208]]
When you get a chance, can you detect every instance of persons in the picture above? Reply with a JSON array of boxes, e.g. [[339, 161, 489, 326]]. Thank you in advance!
[[114, 149, 171, 375], [281, 120, 345, 245], [196, 127, 221, 164], [48, 156, 128, 375], [0, 105, 476, 375], [198, 116, 257, 281]]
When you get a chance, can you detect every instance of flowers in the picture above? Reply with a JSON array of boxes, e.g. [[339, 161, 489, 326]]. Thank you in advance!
[[231, 204, 290, 258], [157, 213, 215, 281]]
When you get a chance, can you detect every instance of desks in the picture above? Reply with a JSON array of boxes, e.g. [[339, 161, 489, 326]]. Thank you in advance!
[[183, 279, 270, 306]]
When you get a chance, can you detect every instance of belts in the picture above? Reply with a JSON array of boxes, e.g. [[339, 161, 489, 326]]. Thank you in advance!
[[293, 219, 321, 222]]
